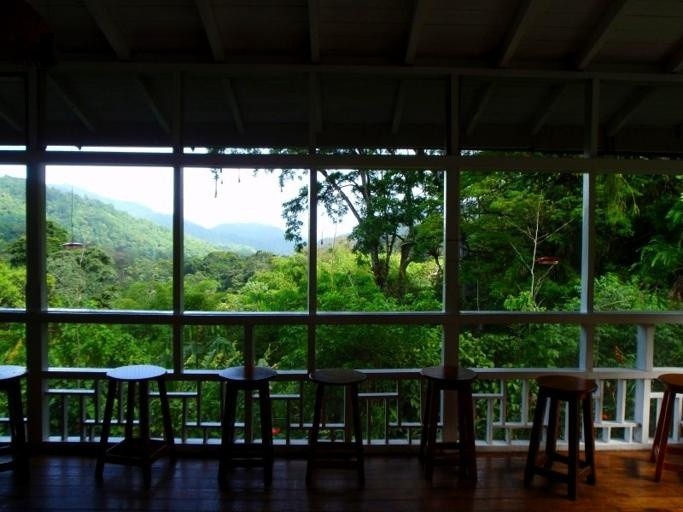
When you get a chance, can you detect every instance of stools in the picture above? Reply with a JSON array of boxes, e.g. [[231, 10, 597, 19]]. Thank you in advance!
[[94, 364, 176, 492], [417, 366, 481, 486], [0, 364, 30, 484], [650, 373, 682, 482], [306, 367, 368, 491], [523, 375, 598, 500], [217, 365, 277, 493]]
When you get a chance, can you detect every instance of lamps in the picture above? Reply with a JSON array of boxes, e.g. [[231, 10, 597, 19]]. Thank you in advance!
[[537, 257, 559, 265], [62, 242, 84, 250]]
